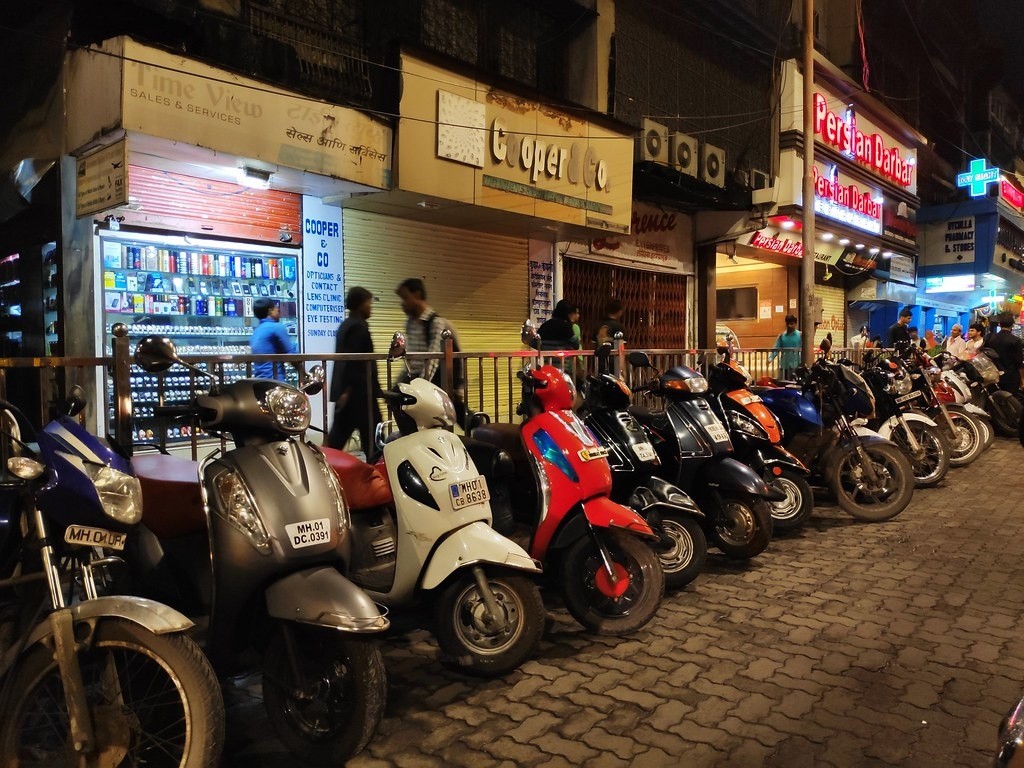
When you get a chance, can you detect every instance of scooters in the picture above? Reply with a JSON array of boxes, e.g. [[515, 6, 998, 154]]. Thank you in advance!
[[307, 318, 814, 677], [131, 334, 390, 768]]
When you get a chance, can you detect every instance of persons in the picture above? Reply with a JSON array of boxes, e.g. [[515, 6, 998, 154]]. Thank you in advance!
[[592, 298, 629, 385], [850, 325, 882, 369], [766, 314, 802, 380], [329, 286, 385, 457], [532, 299, 584, 392], [393, 278, 446, 435], [983, 314, 1024, 436], [888, 309, 912, 348], [909, 326, 944, 350], [942, 324, 986, 360], [249, 298, 312, 382]]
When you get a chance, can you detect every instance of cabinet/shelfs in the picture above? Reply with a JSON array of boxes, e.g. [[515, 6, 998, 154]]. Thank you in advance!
[[99, 236, 298, 450]]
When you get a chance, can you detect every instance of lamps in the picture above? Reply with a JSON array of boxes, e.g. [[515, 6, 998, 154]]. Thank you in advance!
[[238, 167, 270, 190], [872, 189, 884, 205]]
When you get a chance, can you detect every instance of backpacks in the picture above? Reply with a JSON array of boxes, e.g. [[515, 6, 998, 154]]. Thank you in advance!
[[424, 315, 462, 389]]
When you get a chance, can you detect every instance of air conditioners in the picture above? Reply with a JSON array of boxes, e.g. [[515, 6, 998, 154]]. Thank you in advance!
[[634, 118, 668, 167], [698, 143, 726, 189], [668, 131, 698, 178]]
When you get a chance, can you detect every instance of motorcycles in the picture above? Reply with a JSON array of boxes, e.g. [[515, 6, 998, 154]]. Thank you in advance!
[[0, 386, 224, 768], [750, 332, 1024, 522]]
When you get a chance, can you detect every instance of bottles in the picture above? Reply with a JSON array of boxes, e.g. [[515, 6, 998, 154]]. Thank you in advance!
[[122, 246, 282, 279], [154, 294, 236, 317]]
